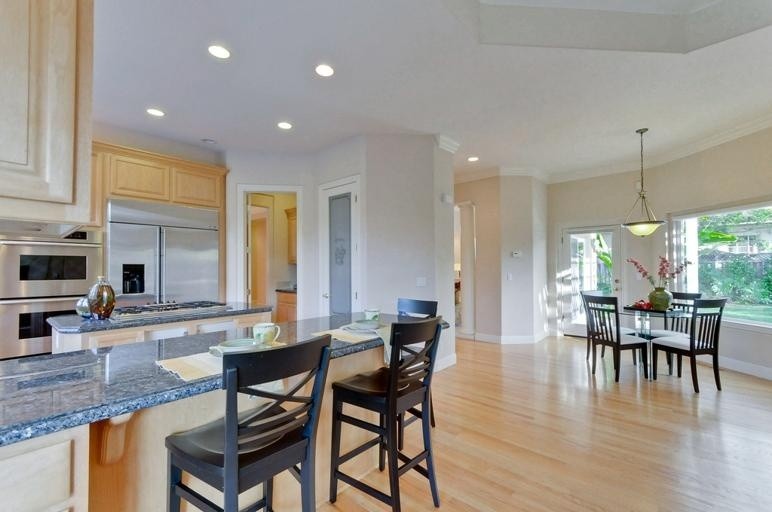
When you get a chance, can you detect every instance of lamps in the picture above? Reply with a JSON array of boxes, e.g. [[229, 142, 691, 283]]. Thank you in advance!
[[621, 128, 664, 237]]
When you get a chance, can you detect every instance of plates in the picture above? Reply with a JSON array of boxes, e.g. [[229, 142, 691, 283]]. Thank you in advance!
[[219, 339, 253, 349]]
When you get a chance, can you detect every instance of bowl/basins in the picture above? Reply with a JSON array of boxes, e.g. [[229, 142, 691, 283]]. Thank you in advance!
[[356, 321, 378, 330]]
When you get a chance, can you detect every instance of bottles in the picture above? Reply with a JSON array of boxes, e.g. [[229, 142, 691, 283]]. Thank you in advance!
[[88, 276, 116, 319]]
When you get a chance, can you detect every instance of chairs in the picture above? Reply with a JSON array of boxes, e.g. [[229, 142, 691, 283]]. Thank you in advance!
[[331, 318, 442, 512], [651, 298, 727, 393], [638, 292, 702, 365], [580, 290, 638, 366], [583, 295, 648, 382], [165, 334, 331, 512]]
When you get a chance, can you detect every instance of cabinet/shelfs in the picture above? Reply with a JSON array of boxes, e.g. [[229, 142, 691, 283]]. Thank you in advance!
[[92, 139, 229, 305], [47, 300, 273, 356]]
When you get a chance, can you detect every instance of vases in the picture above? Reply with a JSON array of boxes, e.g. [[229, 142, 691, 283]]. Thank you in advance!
[[648, 287, 671, 311]]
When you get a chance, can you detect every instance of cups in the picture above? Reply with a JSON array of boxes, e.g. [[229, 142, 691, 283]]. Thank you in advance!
[[253, 323, 280, 344], [364, 309, 378, 320]]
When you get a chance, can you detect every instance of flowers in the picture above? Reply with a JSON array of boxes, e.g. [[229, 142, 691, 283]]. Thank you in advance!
[[625, 255, 692, 287]]
